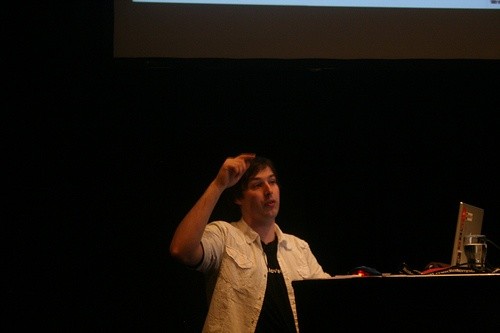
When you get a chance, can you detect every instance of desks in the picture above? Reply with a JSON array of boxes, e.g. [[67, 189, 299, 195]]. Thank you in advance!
[[291, 271, 500, 333]]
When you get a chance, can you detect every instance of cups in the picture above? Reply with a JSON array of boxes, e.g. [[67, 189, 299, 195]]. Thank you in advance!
[[462, 234, 487, 272]]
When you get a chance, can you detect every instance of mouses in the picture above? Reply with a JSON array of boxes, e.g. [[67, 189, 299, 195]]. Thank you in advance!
[[353, 267, 382, 276]]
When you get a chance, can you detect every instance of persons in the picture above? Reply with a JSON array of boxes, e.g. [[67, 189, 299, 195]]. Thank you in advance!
[[170, 153, 330, 333]]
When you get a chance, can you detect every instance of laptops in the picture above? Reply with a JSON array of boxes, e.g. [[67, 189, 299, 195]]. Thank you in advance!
[[434, 202, 484, 274]]
